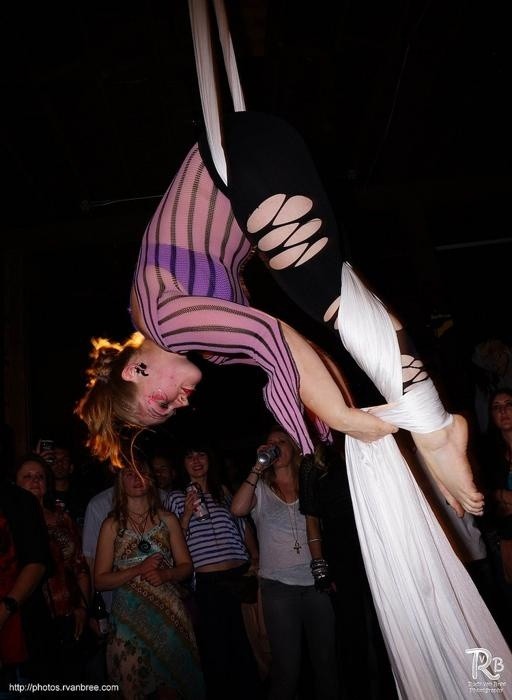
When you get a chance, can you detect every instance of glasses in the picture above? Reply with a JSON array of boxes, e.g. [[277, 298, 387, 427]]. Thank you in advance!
[[23, 473, 45, 481]]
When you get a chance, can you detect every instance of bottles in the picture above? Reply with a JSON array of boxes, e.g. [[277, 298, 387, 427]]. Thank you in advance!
[[94, 588, 110, 635]]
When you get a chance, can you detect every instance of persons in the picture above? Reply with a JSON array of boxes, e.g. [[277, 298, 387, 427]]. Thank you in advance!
[[0, 340, 512, 700], [71, 109, 488, 524]]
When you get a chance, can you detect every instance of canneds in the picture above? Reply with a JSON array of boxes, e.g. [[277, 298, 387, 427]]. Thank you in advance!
[[40, 440, 55, 464], [186, 481, 211, 522], [259, 445, 279, 464]]
